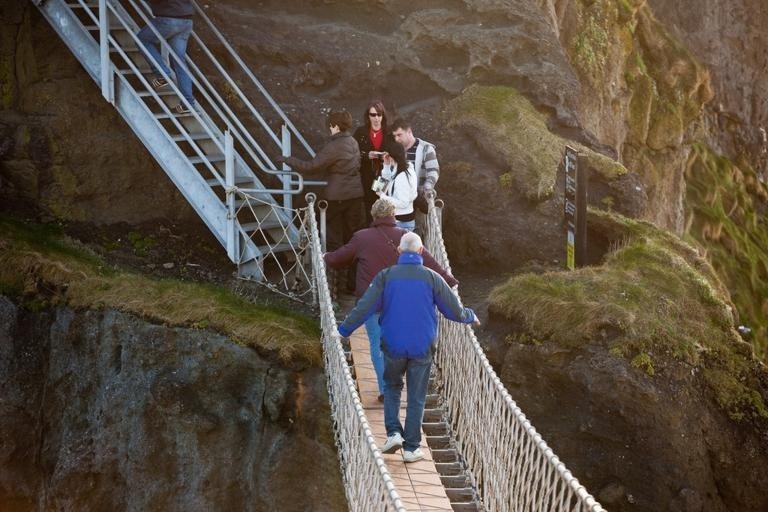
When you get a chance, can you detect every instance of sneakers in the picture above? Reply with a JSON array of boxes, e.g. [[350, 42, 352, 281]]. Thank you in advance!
[[377, 393, 385, 402], [381, 431, 405, 455], [402, 447, 426, 463], [170, 100, 197, 114], [148, 76, 172, 90]]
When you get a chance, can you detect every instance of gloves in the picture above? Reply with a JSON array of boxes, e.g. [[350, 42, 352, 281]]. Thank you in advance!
[[269, 154, 289, 165]]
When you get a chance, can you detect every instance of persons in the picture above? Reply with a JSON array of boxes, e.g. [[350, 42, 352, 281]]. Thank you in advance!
[[329, 230, 480, 465], [387, 115, 439, 218], [317, 196, 459, 403], [270, 110, 367, 295], [369, 139, 420, 234], [351, 97, 394, 230], [135, 0, 198, 114]]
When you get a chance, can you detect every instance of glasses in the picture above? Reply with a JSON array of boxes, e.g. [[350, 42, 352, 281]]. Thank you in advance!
[[367, 111, 383, 118]]
